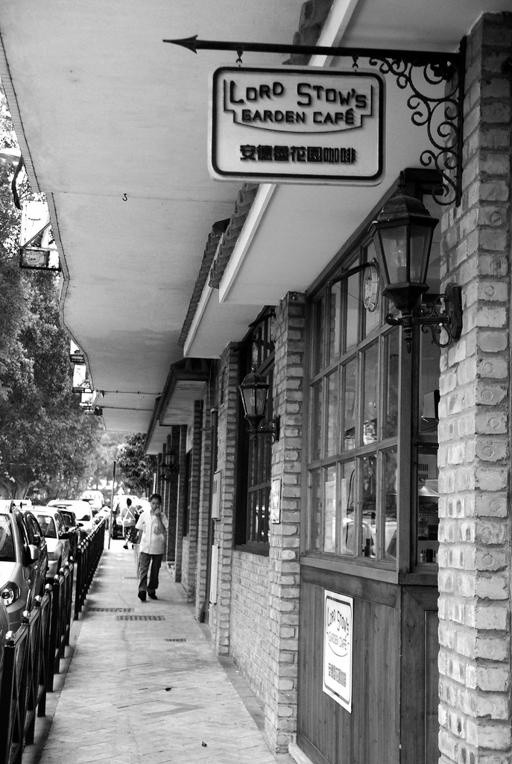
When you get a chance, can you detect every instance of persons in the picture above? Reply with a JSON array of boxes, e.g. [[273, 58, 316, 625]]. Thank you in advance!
[[121, 497, 138, 549], [134, 493, 170, 602]]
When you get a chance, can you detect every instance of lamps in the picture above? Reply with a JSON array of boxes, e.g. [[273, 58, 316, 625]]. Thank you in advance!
[[366, 182, 464, 348], [239, 365, 280, 442]]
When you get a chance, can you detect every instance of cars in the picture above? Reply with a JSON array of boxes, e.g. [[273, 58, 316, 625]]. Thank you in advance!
[[2, 480, 109, 624], [112, 494, 142, 541]]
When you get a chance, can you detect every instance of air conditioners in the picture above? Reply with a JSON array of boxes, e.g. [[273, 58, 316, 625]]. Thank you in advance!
[[69, 340, 101, 416], [19, 199, 50, 250]]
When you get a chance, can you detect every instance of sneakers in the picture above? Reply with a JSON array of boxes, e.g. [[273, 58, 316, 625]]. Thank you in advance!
[[148, 592, 157, 599], [123, 546, 128, 549], [138, 591, 146, 601]]
